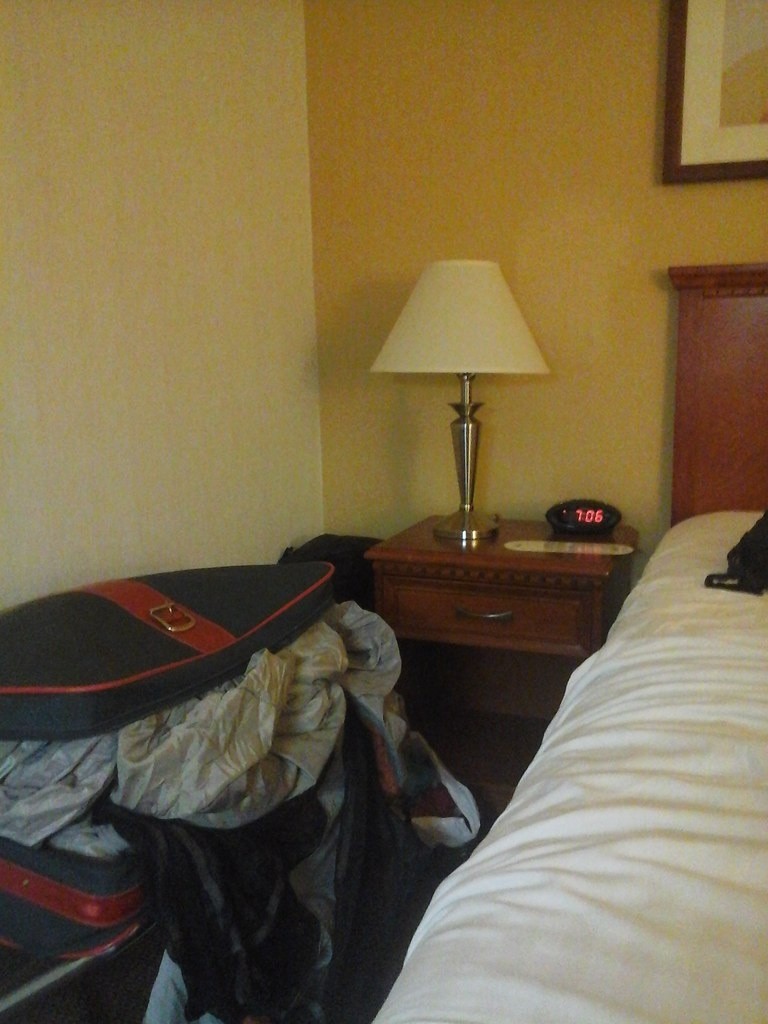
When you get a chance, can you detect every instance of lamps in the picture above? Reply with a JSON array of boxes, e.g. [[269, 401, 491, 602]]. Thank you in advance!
[[367, 258, 551, 540]]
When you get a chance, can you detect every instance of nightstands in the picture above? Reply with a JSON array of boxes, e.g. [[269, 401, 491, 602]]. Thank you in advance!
[[364, 516, 641, 828]]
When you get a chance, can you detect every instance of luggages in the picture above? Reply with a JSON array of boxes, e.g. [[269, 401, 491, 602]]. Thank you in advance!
[[0, 560, 408, 962]]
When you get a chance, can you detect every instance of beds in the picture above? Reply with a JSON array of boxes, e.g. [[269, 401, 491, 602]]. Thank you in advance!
[[369, 263, 768, 1024]]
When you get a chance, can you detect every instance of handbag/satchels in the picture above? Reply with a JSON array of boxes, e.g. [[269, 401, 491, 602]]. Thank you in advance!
[[704, 509, 768, 596]]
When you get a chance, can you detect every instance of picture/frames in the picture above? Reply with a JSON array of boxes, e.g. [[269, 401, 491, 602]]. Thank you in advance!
[[662, 0, 768, 185]]
